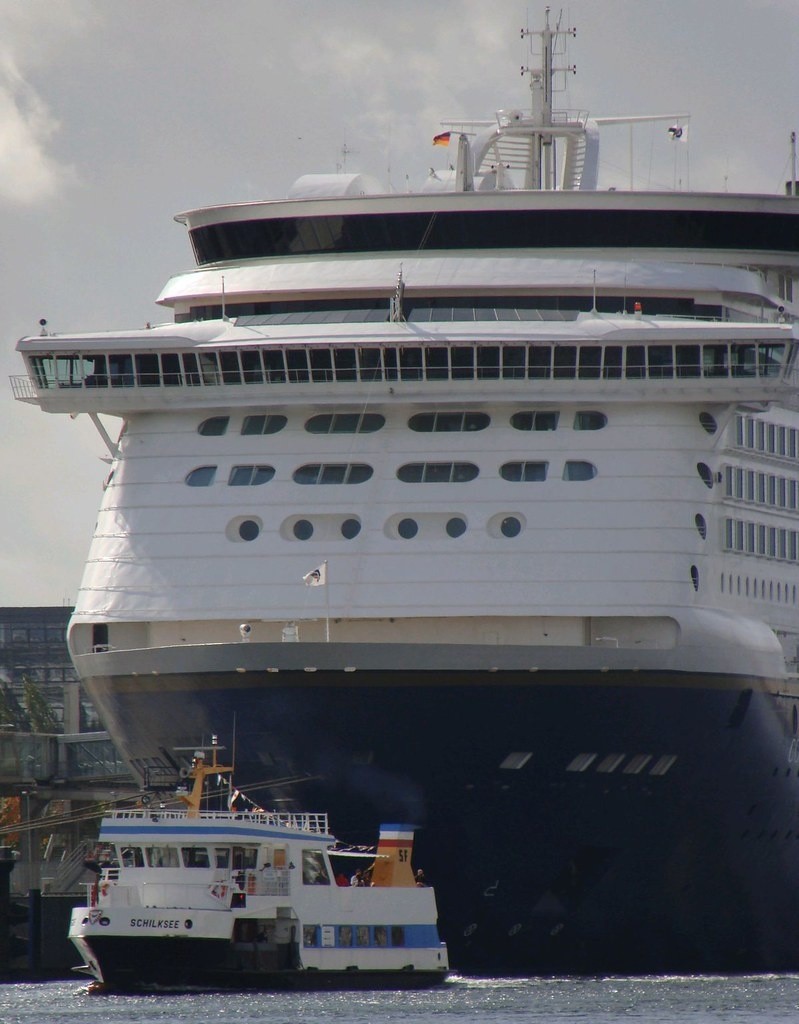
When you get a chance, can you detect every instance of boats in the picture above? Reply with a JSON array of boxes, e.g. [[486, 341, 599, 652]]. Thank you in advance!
[[9, 4, 799, 981], [65, 735, 447, 994]]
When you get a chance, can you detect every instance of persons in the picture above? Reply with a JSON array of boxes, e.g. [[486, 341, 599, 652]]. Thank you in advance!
[[337, 869, 371, 886], [415, 869, 426, 887]]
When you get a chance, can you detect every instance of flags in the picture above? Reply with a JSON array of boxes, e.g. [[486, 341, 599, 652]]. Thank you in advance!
[[303, 564, 325, 587], [668, 124, 688, 143], [433, 131, 450, 147]]
